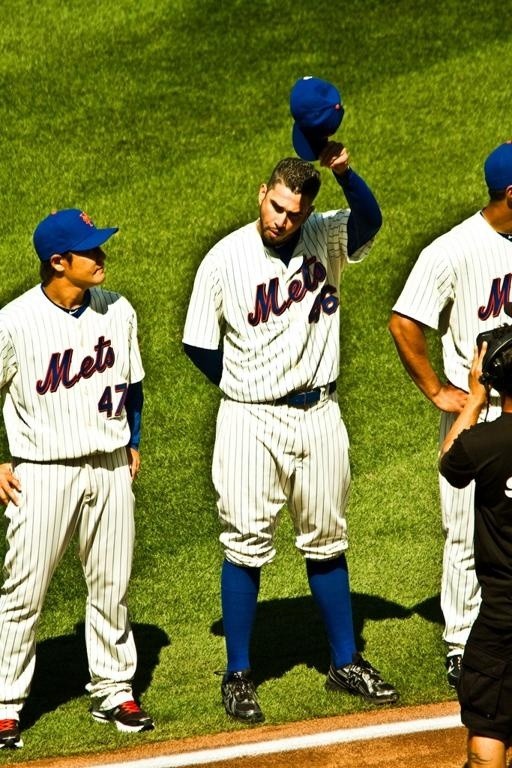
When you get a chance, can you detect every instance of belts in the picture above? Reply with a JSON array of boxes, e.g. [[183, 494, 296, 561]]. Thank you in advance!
[[282, 381, 337, 407]]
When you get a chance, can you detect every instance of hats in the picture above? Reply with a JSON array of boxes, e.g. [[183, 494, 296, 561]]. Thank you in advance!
[[34, 209, 120, 262], [484, 141, 512, 189], [290, 75, 344, 161]]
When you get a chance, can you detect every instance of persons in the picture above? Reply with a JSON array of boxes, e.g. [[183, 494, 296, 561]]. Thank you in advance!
[[389, 142, 511, 690], [177, 132, 404, 722], [1, 209, 155, 751], [436, 323, 511, 767]]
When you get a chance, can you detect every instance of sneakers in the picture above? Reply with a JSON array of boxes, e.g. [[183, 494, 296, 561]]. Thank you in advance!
[[91, 700, 154, 732], [326, 653, 399, 704], [0, 719, 24, 750], [221, 671, 264, 722], [446, 649, 463, 686]]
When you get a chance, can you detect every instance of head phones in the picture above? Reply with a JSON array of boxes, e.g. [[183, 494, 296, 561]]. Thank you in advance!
[[479, 337, 512, 390]]
